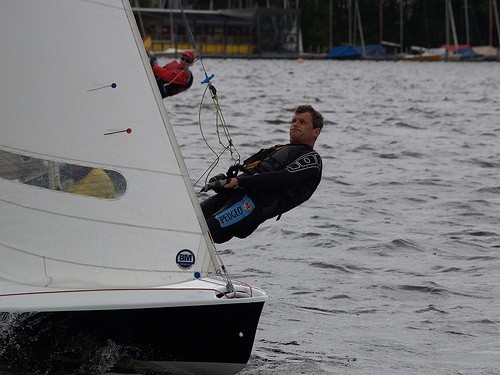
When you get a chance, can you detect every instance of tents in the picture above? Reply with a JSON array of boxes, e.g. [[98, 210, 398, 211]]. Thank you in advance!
[[356, 45, 385, 57], [326, 44, 358, 59], [418, 44, 496, 58]]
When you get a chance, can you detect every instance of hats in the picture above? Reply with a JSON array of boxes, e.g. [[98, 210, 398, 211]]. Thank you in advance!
[[182, 50, 195, 61]]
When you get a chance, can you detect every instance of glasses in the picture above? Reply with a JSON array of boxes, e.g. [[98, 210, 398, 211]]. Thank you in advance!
[[181, 57, 191, 63]]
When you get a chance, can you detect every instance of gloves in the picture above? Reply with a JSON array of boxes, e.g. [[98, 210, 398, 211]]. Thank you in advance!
[[150, 54, 159, 65]]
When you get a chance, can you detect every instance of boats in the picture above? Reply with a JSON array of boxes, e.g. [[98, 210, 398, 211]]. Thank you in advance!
[[324, 37, 500, 61], [0, 1, 267, 374]]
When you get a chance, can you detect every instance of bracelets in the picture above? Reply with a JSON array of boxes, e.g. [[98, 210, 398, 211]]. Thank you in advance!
[[233, 180, 238, 190]]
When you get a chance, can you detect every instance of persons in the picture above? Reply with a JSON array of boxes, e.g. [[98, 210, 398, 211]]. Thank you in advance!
[[64, 163, 114, 199], [148, 50, 194, 99], [199, 105, 324, 244]]
